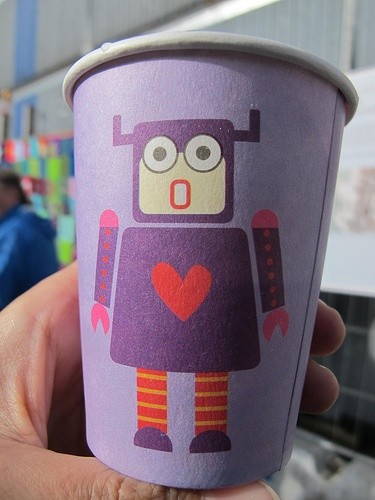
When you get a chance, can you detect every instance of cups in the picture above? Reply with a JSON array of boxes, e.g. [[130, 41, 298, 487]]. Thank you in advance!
[[58, 27, 360, 492]]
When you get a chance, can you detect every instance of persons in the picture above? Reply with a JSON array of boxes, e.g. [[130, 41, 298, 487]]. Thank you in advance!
[[0, 255, 349, 500], [0, 172, 60, 310]]
